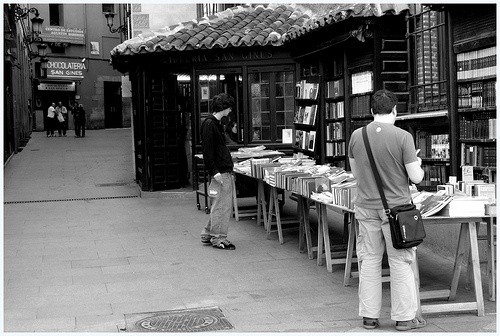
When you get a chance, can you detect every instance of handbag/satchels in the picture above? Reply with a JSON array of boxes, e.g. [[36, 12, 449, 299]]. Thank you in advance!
[[56, 112, 65, 123], [385, 203, 426, 249]]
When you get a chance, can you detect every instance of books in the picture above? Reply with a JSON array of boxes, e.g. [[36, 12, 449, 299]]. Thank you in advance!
[[250, 157, 359, 210], [414, 131, 451, 186], [457, 46, 497, 183], [294, 83, 319, 152], [410, 180, 496, 216], [326, 72, 374, 156]]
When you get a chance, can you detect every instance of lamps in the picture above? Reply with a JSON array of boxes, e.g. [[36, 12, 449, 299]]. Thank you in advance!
[[105, 10, 125, 34], [17, 8, 43, 40], [27, 36, 46, 60], [32, 58, 49, 80]]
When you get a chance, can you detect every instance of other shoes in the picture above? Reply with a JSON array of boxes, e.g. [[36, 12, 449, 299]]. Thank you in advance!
[[200, 238, 212, 246], [395, 317, 427, 330], [212, 240, 235, 250], [361, 317, 379, 329]]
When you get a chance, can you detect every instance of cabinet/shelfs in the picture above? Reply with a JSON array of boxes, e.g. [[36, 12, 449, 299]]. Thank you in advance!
[[178, 44, 496, 188]]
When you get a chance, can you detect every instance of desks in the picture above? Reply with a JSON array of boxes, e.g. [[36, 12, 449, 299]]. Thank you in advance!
[[230, 163, 496, 318]]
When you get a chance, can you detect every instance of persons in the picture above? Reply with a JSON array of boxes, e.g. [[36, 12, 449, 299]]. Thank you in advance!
[[200, 93, 235, 250], [348, 89, 426, 330], [47, 102, 68, 137], [71, 101, 86, 138]]
